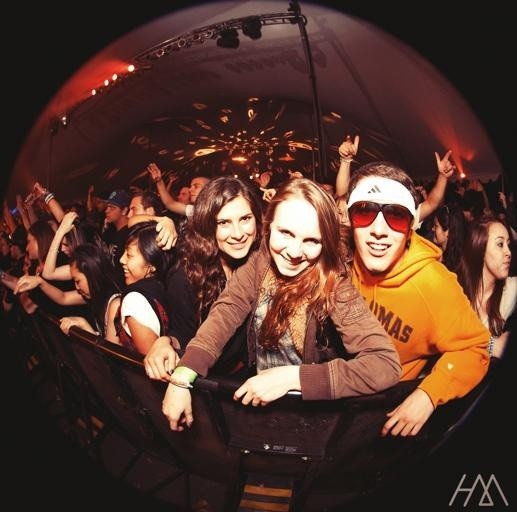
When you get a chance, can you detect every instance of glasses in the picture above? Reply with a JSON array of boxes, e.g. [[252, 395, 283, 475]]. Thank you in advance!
[[348, 200, 414, 234], [439, 203, 450, 216]]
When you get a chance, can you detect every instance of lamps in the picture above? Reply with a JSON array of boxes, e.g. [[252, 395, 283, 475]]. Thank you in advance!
[[47, 16, 262, 136]]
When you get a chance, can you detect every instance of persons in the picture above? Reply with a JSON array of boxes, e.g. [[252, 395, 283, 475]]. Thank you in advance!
[[0, 132, 516, 436]]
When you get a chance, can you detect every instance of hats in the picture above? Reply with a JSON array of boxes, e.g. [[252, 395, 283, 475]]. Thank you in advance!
[[97, 189, 130, 210]]
